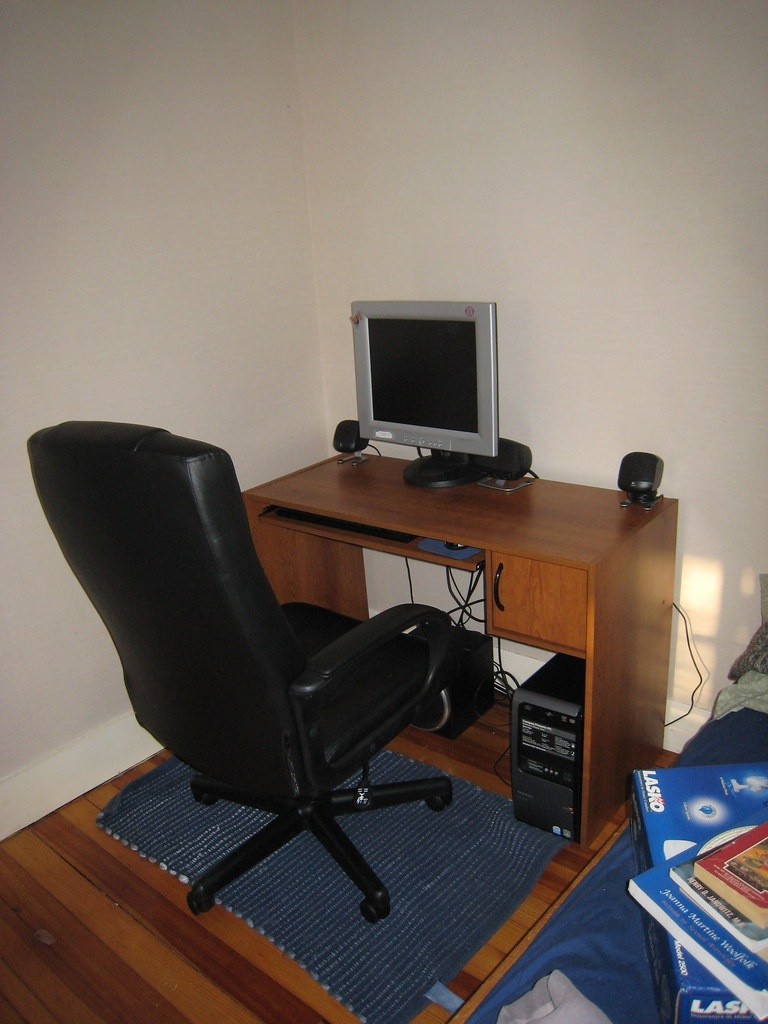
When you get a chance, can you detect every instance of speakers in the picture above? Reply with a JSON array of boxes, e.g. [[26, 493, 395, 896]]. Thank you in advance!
[[333, 420, 369, 466], [408, 624, 494, 740], [470, 437, 533, 481], [618, 452, 664, 510]]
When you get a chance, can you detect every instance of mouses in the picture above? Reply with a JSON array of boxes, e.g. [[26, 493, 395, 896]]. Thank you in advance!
[[444, 540, 467, 550]]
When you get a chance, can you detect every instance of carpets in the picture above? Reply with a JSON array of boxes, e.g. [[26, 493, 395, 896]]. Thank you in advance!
[[97, 750, 570, 1023]]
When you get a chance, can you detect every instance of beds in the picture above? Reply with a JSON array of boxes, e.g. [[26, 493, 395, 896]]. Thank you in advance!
[[468, 579, 768, 1024]]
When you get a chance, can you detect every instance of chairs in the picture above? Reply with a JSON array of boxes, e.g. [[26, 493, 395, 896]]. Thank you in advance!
[[26, 420, 466, 925]]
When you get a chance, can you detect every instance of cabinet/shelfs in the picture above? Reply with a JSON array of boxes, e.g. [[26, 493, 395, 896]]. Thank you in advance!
[[242, 448, 679, 846]]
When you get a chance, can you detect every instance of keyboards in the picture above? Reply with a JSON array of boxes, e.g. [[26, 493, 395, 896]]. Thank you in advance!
[[275, 508, 418, 543]]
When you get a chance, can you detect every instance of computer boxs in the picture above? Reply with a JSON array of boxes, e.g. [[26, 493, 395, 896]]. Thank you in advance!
[[510, 652, 586, 842]]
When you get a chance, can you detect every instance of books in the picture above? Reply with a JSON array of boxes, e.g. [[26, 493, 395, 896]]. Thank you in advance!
[[627, 809, 767, 1021]]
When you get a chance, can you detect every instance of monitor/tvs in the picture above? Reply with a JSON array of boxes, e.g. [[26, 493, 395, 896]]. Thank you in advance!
[[351, 301, 499, 488]]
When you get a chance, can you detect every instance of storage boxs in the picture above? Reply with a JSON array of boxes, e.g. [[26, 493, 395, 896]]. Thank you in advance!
[[627, 763, 768, 1024]]
